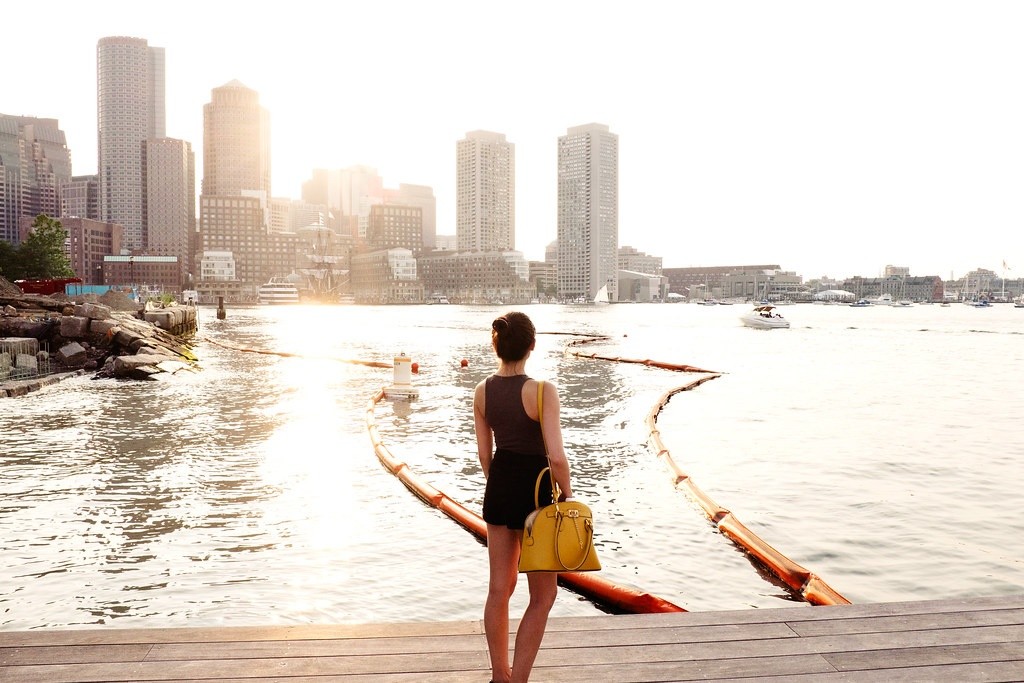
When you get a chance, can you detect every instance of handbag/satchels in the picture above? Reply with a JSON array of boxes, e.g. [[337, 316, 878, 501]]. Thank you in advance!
[[516, 467, 601, 573]]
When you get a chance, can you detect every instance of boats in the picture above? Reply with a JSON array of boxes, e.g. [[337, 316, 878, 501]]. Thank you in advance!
[[940, 303, 951, 307], [920, 299, 934, 306], [258, 275, 300, 305], [849, 300, 875, 307], [735, 298, 789, 330], [1014, 298, 1024, 308]]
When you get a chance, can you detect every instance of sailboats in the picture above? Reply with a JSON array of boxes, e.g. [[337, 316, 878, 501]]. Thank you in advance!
[[961, 271, 1008, 307], [888, 272, 914, 307], [696, 274, 733, 305]]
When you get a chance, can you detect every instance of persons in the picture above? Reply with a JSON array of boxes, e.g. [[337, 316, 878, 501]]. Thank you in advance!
[[474, 312, 573, 683]]
